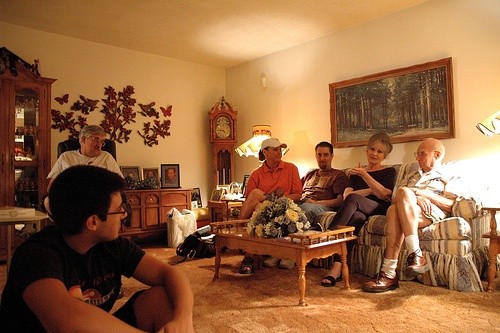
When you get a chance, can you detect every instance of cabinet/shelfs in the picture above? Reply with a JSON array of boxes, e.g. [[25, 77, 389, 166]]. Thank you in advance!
[[118, 189, 192, 241], [0, 46, 59, 264]]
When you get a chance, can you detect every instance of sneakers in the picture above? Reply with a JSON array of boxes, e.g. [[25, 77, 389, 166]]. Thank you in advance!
[[262, 255, 278, 267], [279, 259, 297, 269]]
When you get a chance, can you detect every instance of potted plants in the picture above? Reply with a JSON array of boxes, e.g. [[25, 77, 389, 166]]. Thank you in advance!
[[245, 198, 311, 238]]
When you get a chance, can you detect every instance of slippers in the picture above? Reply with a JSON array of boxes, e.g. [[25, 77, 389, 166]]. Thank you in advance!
[[321, 274, 344, 287]]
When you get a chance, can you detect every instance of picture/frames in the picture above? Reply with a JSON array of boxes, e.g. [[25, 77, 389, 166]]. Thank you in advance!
[[120, 166, 142, 182], [161, 163, 181, 189], [208, 174, 257, 203], [328, 56, 455, 148], [191, 187, 203, 208], [143, 167, 159, 189]]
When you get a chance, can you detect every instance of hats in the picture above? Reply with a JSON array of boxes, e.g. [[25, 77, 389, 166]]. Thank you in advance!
[[262, 138, 288, 151]]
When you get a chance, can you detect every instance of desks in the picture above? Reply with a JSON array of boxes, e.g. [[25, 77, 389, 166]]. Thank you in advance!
[[0, 206, 49, 275]]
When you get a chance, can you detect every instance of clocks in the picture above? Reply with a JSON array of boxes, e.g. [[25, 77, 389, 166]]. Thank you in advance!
[[208, 96, 238, 196]]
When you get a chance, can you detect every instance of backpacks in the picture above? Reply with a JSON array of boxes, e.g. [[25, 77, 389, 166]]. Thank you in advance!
[[175, 226, 211, 252], [188, 234, 216, 260]]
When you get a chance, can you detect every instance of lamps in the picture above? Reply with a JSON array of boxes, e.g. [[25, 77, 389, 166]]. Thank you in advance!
[[475, 109, 500, 136], [233, 124, 290, 168]]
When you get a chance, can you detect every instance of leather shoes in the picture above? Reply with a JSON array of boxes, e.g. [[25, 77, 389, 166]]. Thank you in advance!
[[362, 274, 398, 292], [405, 253, 429, 278]]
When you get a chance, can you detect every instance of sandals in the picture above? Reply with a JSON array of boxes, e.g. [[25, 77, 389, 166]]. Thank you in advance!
[[239, 258, 256, 274]]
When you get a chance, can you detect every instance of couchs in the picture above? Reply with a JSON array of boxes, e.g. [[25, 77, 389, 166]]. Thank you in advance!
[[307, 194, 492, 293]]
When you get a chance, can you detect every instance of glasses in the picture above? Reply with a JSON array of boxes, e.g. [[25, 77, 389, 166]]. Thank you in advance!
[[87, 134, 107, 146], [414, 150, 441, 157], [95, 202, 130, 217]]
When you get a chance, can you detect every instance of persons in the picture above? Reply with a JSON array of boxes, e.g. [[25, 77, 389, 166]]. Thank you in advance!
[[146, 170, 155, 177], [263, 140, 348, 272], [360, 137, 463, 293], [166, 167, 176, 183], [0, 164, 195, 333], [127, 171, 134, 178], [44, 124, 125, 217], [320, 132, 398, 287], [208, 134, 303, 274]]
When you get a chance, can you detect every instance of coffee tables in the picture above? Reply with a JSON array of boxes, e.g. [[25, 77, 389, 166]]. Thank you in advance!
[[209, 218, 358, 305]]
